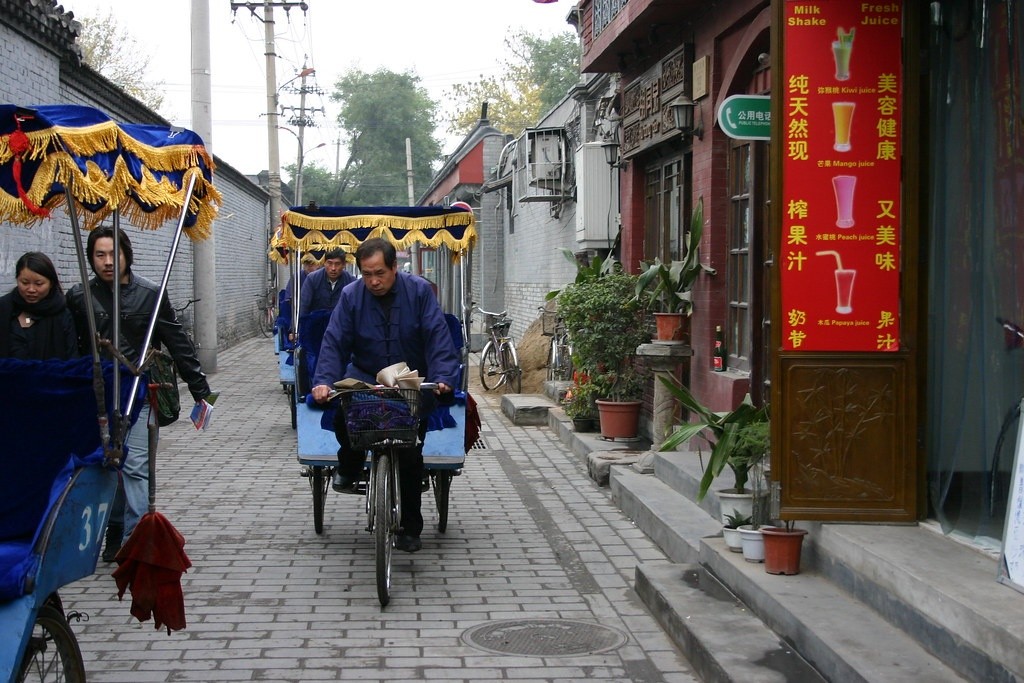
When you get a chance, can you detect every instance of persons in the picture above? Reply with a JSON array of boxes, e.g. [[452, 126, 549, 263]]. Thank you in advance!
[[284, 260, 317, 303], [0, 252, 79, 362], [289, 246, 357, 341], [312, 237, 461, 552], [67, 225, 212, 560]]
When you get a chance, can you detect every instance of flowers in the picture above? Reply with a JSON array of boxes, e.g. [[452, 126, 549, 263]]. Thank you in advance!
[[567, 374, 594, 416]]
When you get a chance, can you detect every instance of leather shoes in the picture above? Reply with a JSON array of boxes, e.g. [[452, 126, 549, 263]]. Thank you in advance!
[[400, 535, 421, 551], [332, 468, 355, 489]]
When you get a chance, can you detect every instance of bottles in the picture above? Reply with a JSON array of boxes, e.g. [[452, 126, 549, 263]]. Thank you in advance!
[[713, 325, 726, 372]]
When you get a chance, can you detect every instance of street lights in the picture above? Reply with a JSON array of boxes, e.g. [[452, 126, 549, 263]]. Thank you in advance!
[[294, 142, 325, 205]]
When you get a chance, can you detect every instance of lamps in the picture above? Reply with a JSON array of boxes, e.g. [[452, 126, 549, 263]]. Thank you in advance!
[[668, 92, 707, 142], [601, 133, 629, 173]]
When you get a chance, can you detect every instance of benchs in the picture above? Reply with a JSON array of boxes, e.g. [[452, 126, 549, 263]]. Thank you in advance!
[[277, 289, 298, 353], [0, 356, 149, 606], [301, 306, 468, 408]]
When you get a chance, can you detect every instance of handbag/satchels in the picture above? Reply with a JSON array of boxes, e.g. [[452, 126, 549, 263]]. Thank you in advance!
[[144, 350, 182, 425]]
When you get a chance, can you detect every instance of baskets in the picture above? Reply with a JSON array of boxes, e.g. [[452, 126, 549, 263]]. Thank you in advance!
[[340, 388, 423, 448]]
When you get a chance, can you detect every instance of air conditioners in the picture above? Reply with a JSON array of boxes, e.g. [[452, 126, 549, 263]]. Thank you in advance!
[[530, 135, 560, 183]]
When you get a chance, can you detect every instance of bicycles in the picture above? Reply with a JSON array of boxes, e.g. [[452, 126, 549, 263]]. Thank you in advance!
[[470, 308, 523, 394], [254, 288, 276, 339], [170, 299, 202, 341], [537, 306, 574, 381]]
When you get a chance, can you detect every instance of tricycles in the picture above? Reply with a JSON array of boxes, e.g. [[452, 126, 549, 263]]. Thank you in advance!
[[0, 105, 221, 683], [264, 201, 469, 605]]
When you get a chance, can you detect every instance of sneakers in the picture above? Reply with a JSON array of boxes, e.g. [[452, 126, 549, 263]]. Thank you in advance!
[[102, 528, 124, 561]]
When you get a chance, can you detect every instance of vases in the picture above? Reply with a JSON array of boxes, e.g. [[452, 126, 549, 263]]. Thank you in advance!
[[758, 528, 809, 577], [571, 417, 596, 431]]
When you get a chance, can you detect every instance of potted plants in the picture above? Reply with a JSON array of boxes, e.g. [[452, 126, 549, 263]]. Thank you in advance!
[[559, 275, 656, 437], [634, 194, 717, 341], [656, 368, 768, 553], [737, 421, 771, 565]]
